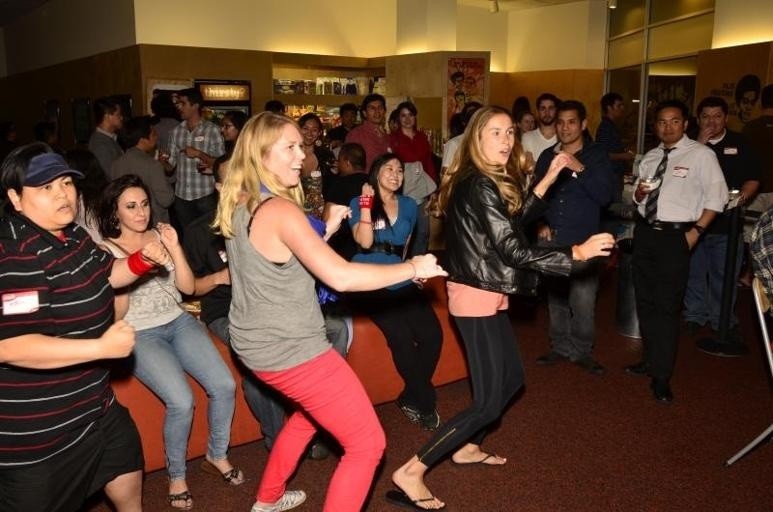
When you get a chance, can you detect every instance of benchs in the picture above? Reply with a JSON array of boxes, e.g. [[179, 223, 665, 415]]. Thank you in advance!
[[95, 302, 473, 479]]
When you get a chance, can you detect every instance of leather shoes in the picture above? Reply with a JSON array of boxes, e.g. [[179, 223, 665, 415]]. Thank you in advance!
[[626, 364, 672, 403]]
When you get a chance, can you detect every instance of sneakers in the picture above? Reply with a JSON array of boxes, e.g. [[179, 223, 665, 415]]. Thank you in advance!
[[251, 490, 307, 512], [396, 391, 440, 431]]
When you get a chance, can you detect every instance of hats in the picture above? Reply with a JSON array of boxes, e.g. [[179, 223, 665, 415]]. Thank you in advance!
[[21, 152, 84, 187]]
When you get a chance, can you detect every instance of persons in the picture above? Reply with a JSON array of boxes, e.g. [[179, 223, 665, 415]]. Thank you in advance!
[[728, 74, 761, 132], [447, 72, 483, 113], [1, 87, 773, 512]]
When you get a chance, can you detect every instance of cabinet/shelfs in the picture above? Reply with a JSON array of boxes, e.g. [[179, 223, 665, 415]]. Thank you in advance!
[[265, 61, 386, 129]]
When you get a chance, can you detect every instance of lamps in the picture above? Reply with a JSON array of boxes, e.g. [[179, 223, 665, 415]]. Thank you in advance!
[[607, 1, 618, 9], [487, 1, 500, 15]]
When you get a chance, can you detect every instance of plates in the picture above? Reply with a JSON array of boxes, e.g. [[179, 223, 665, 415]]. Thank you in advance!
[[185, 300, 201, 313]]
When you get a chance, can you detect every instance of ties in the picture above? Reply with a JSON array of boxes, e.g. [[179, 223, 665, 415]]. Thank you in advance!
[[645, 148, 675, 225]]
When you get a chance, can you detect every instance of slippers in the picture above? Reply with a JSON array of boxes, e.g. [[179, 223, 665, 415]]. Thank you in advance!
[[201, 461, 244, 487], [166, 491, 193, 510], [451, 454, 504, 465], [387, 491, 446, 512]]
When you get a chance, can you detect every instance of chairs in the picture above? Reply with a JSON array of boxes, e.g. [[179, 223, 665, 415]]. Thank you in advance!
[[724, 272, 770, 467]]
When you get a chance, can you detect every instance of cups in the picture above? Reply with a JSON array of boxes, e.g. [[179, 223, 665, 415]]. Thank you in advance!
[[728, 186, 740, 194], [642, 176, 658, 185]]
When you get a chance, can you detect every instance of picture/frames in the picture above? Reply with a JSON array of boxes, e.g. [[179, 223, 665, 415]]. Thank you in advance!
[[147, 76, 192, 115]]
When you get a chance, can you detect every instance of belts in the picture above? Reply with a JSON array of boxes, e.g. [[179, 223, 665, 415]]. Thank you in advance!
[[648, 221, 693, 230]]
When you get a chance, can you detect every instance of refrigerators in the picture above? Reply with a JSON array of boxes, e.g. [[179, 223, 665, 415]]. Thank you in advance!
[[192, 80, 250, 121]]
